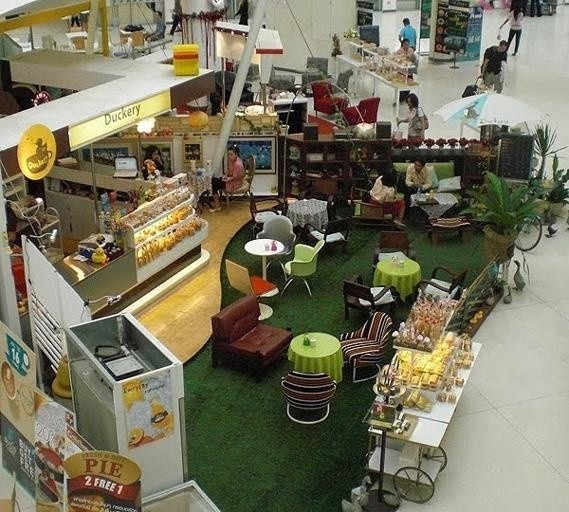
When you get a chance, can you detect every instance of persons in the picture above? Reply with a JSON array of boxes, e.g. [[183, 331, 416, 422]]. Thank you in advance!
[[70, 13, 80, 28], [142, 145, 164, 182], [530, 0, 542, 17], [480, 40, 508, 93], [405, 158, 434, 215], [369, 169, 407, 227], [499, 5, 524, 56], [146, 11, 166, 43], [208, 144, 245, 213], [206, 92, 223, 117], [397, 39, 414, 79], [397, 94, 426, 141], [398, 18, 417, 51], [234, 0, 248, 26], [169, 6, 182, 36]]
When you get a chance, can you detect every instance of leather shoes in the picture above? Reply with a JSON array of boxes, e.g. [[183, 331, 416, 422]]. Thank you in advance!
[[209, 206, 222, 213], [512, 53, 517, 57], [393, 219, 406, 227]]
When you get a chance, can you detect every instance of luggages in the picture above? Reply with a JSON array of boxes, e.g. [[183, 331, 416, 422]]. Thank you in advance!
[[461, 76, 484, 98]]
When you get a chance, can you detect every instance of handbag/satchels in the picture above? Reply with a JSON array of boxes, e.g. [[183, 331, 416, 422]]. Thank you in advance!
[[412, 107, 429, 131]]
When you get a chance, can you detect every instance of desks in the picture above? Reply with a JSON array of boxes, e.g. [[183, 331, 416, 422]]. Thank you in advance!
[[286, 199, 329, 234], [244, 238, 284, 281], [409, 193, 463, 223], [372, 257, 422, 302], [287, 331, 345, 385], [258, 88, 314, 131]]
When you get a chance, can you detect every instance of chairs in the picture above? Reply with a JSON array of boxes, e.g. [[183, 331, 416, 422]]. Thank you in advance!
[[118, 31, 131, 58], [301, 56, 329, 86], [342, 272, 398, 326], [220, 154, 255, 208], [331, 69, 353, 95], [269, 64, 294, 91], [343, 97, 380, 124], [249, 191, 284, 239], [281, 371, 337, 425], [414, 264, 468, 302], [71, 35, 88, 54], [224, 259, 279, 322], [304, 217, 352, 255], [372, 229, 416, 269], [255, 215, 296, 282], [340, 310, 392, 384], [311, 80, 348, 115], [147, 25, 166, 54], [277, 239, 327, 296], [210, 69, 253, 114], [130, 32, 144, 59], [349, 184, 384, 228]]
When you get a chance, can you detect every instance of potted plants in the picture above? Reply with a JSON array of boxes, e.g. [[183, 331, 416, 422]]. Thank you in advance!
[[456, 170, 551, 265], [545, 153, 569, 215]]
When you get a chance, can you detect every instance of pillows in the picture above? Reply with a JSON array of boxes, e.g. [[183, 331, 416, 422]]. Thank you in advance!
[[427, 167, 439, 188], [437, 176, 461, 192]]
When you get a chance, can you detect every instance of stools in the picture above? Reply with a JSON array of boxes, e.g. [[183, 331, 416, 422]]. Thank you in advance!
[[427, 215, 473, 248]]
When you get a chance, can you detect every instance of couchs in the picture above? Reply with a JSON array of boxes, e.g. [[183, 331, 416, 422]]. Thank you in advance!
[[390, 163, 462, 209], [212, 295, 291, 369]]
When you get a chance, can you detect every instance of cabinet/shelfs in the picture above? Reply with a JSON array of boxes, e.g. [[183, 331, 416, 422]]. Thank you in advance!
[[361, 296, 482, 424], [420, 0, 482, 58], [45, 158, 171, 237], [65, 311, 188, 499], [108, 184, 208, 284], [335, 36, 419, 123], [53, 240, 137, 314], [282, 131, 497, 203]]
